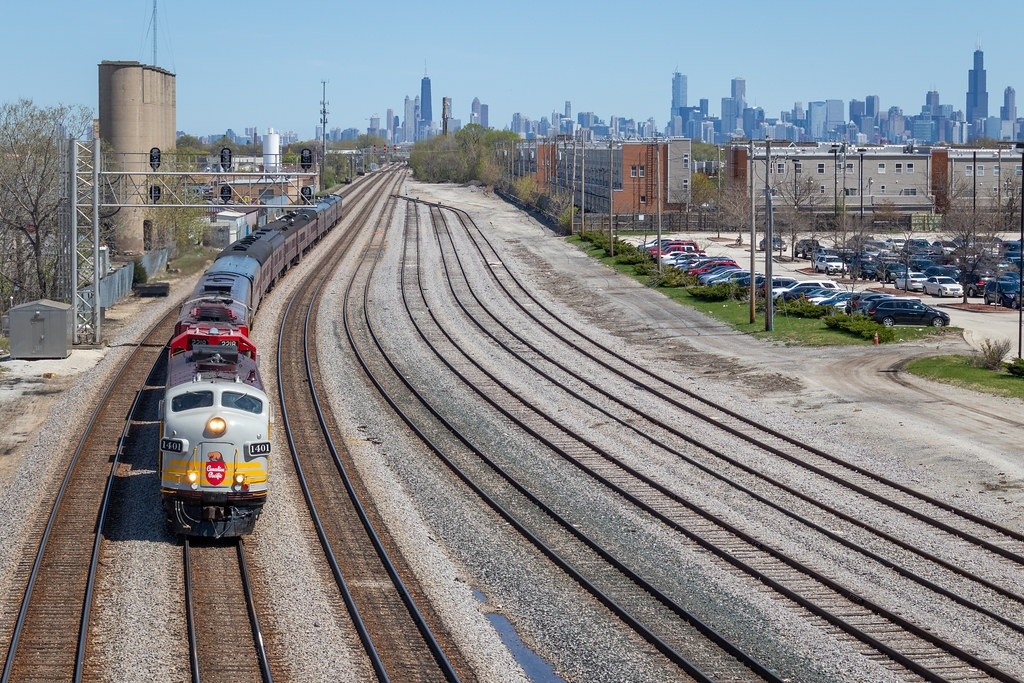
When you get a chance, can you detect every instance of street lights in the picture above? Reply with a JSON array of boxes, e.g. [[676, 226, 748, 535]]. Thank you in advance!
[[832, 144, 840, 216], [791, 159, 799, 209], [858, 148, 867, 223], [518, 150, 524, 179], [557, 150, 568, 202], [529, 150, 534, 179], [502, 150, 507, 180]]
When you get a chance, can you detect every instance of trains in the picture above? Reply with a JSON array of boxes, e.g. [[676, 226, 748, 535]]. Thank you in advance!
[[156, 193, 345, 538]]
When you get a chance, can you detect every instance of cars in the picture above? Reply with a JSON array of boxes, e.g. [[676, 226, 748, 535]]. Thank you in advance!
[[883, 263, 912, 283], [922, 275, 964, 298], [849, 233, 1024, 279], [984, 280, 1020, 307], [894, 272, 928, 291], [637, 237, 848, 314]]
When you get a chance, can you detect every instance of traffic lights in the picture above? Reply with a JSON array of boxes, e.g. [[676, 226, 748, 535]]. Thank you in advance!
[[373, 144, 376, 153], [393, 145, 396, 153], [383, 144, 386, 153]]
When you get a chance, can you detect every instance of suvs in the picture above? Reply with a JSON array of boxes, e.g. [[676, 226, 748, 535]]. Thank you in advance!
[[846, 291, 895, 316], [924, 266, 960, 281], [956, 270, 995, 297], [864, 297, 949, 329]]
